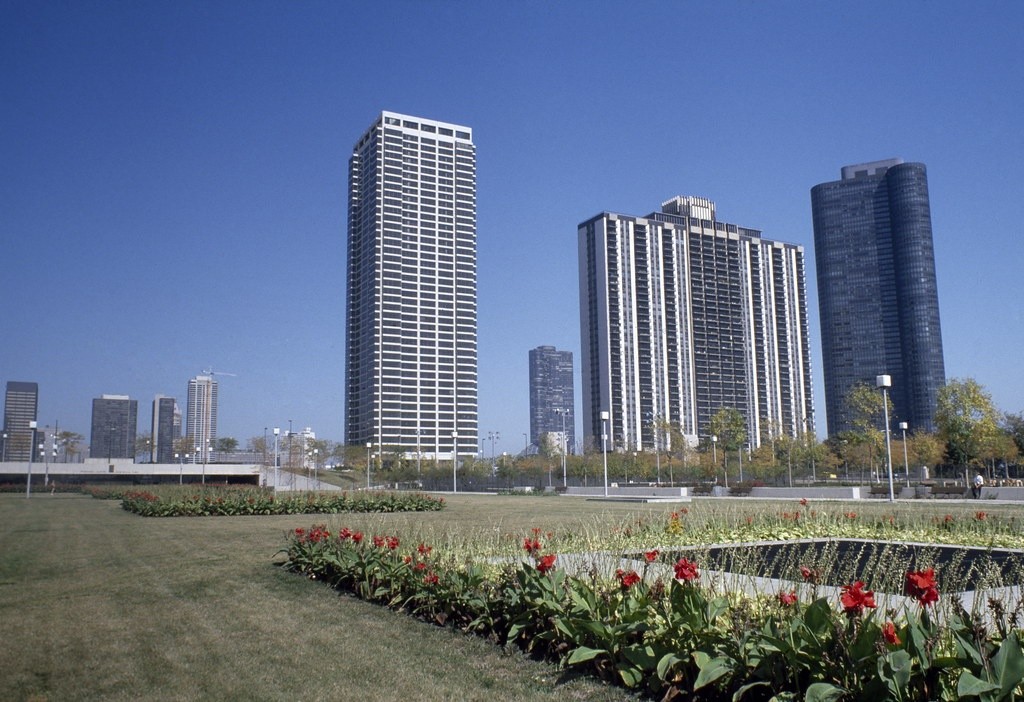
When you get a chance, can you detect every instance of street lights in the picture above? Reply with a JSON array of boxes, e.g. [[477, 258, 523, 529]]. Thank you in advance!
[[876, 374, 895, 501], [270, 427, 280, 495], [366, 442, 371, 490], [598, 411, 609, 498], [313, 448, 319, 492], [23, 421, 37, 497], [195, 438, 213, 486], [553, 408, 569, 487], [307, 452, 312, 495], [481, 439, 486, 459], [174, 453, 189, 486], [487, 431, 499, 491], [502, 452, 507, 468], [898, 422, 910, 487], [287, 419, 293, 469], [523, 432, 527, 461], [263, 427, 268, 466], [710, 435, 718, 483], [2, 433, 9, 462]]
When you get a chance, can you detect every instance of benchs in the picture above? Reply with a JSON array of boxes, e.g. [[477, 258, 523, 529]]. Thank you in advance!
[[868, 487, 902, 498], [690, 488, 713, 495], [554, 487, 568, 494], [533, 486, 545, 492], [727, 487, 752, 496], [929, 487, 967, 498]]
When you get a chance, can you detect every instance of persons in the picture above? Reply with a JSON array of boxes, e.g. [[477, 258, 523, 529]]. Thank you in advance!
[[971, 470, 984, 499]]
